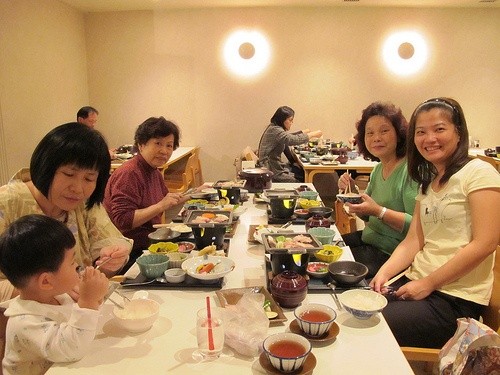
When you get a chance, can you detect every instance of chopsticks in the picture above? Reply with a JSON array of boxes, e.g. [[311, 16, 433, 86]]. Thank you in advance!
[[345, 169, 351, 194], [351, 133, 353, 150]]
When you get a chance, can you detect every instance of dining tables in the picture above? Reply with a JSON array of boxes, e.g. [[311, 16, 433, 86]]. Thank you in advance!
[[468, 148, 500, 164], [109, 146, 194, 223], [43, 182, 416, 375], [290, 146, 379, 184]]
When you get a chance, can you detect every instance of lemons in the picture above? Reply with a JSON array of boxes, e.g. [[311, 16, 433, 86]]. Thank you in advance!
[[276, 236, 286, 242]]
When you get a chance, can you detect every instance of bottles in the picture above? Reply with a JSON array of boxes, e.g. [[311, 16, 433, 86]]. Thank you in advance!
[[270, 269, 308, 309], [293, 185, 313, 205], [305, 214, 330, 232], [326, 138, 330, 145], [301, 147, 311, 157], [338, 153, 348, 164]]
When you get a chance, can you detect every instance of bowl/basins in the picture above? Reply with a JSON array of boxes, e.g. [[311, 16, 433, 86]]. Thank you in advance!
[[263, 191, 388, 374], [113, 188, 248, 333], [336, 193, 362, 205], [304, 147, 359, 164]]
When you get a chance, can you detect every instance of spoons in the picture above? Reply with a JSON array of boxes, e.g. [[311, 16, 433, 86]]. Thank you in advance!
[[122, 278, 168, 286], [328, 282, 343, 310]]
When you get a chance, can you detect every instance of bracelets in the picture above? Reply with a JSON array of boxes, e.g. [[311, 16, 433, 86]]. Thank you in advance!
[[377, 207, 387, 221]]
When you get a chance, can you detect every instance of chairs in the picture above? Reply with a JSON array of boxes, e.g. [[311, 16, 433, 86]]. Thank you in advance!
[[334, 201, 356, 235], [166, 147, 203, 193], [400, 245, 500, 375]]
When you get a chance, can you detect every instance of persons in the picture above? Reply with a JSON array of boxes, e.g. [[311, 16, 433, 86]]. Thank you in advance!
[[77, 106, 117, 160], [369, 98, 500, 349], [337, 102, 421, 280], [102, 117, 192, 275], [0, 122, 135, 358], [258, 106, 322, 183], [0, 214, 109, 375]]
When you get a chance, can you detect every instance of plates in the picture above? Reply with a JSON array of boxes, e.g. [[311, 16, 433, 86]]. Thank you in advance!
[[264, 233, 324, 253], [248, 224, 284, 244], [260, 350, 317, 375], [184, 208, 233, 228], [289, 319, 340, 342], [216, 285, 287, 322], [111, 154, 133, 158], [321, 161, 338, 165], [212, 180, 246, 188], [263, 189, 300, 199]]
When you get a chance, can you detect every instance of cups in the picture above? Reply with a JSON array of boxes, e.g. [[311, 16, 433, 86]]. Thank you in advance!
[[196, 307, 227, 361]]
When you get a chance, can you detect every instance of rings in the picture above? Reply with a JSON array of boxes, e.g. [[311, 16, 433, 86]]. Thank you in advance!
[[401, 294, 405, 301]]
